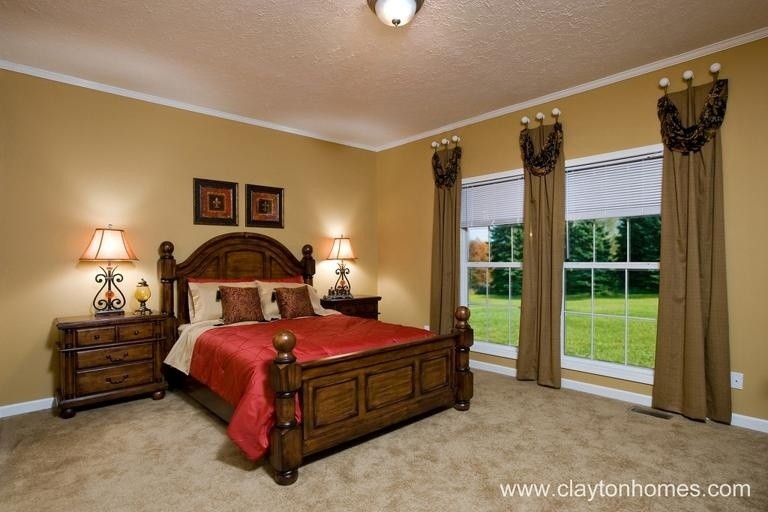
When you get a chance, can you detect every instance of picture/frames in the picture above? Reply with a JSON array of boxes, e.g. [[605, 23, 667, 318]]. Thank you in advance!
[[245, 183, 284, 228], [193, 177, 240, 226]]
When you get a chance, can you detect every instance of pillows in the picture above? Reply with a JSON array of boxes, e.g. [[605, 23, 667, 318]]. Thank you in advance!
[[274, 285, 316, 320], [219, 286, 266, 324], [187, 275, 304, 284], [188, 281, 256, 322], [255, 279, 321, 321]]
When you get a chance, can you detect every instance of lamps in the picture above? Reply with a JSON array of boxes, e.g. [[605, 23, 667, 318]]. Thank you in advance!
[[326, 234, 359, 299], [366, 0, 424, 30], [78, 224, 140, 317], [133, 278, 153, 317]]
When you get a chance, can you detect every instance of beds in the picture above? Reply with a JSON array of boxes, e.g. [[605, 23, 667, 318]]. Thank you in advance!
[[158, 231, 474, 485]]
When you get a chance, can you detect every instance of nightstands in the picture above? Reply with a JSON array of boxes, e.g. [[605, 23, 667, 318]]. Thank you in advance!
[[55, 309, 169, 419], [320, 294, 382, 321]]
